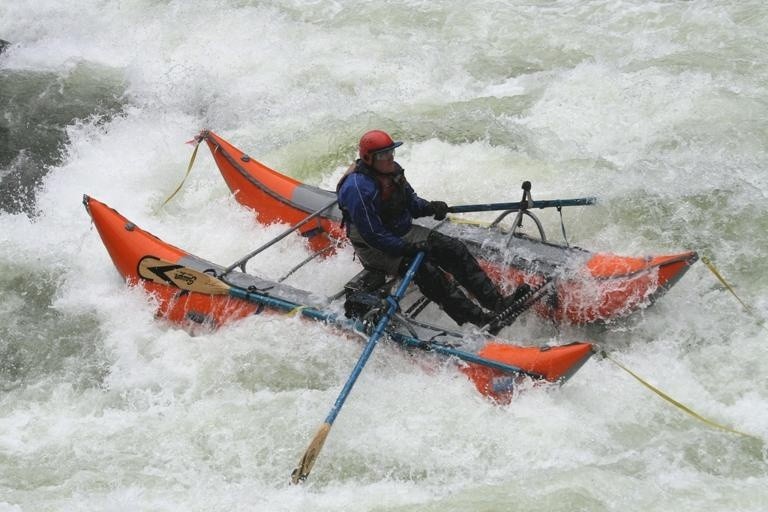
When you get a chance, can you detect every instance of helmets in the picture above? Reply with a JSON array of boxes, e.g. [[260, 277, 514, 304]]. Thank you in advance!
[[358, 130, 403, 166]]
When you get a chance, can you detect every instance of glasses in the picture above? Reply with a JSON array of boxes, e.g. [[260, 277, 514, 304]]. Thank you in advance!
[[373, 149, 394, 160]]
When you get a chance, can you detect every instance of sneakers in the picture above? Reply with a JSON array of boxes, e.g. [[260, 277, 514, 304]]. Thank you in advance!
[[482, 312, 500, 336], [502, 283, 530, 326]]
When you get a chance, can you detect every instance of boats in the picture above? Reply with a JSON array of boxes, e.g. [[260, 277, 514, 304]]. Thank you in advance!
[[82, 129, 698, 405]]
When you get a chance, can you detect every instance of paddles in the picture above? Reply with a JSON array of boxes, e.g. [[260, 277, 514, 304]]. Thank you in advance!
[[137, 255, 545, 380], [290, 249, 428, 480]]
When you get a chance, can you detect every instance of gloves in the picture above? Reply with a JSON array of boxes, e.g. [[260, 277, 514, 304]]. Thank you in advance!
[[429, 200, 446, 220], [405, 240, 432, 254]]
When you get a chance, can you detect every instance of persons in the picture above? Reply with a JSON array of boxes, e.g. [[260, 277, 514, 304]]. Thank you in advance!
[[335, 129, 531, 340]]
